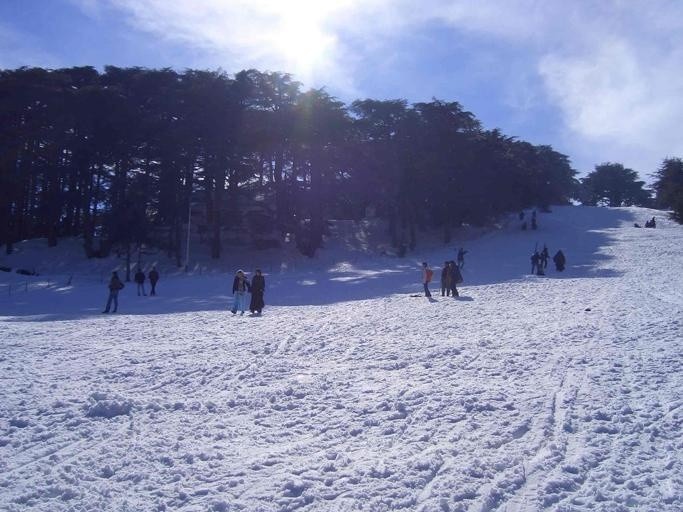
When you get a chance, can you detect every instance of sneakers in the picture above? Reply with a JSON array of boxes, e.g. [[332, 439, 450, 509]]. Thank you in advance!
[[138, 294, 158, 296], [101, 310, 117, 313], [231, 310, 245, 314]]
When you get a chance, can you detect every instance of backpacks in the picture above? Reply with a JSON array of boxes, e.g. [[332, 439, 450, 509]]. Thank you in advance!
[[426, 268, 433, 282]]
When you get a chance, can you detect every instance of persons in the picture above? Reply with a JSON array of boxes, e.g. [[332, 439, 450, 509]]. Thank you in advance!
[[441, 260, 463, 297], [135, 266, 159, 296], [102, 271, 125, 313], [531, 248, 565, 274], [231, 268, 265, 315], [457, 247, 470, 269], [519, 210, 537, 231], [421, 262, 434, 297]]
